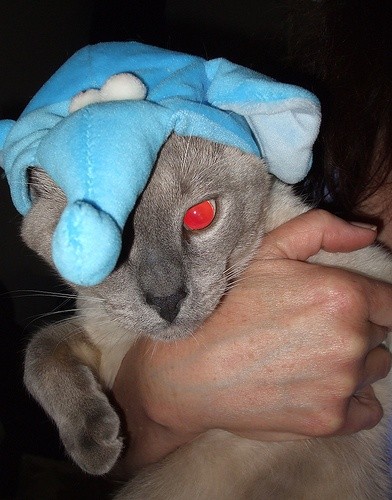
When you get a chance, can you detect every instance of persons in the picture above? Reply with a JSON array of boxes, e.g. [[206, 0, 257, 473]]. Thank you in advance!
[[111, 1, 392, 479]]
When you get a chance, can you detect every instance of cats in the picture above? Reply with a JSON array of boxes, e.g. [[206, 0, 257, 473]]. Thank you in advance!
[[20, 128, 391, 500]]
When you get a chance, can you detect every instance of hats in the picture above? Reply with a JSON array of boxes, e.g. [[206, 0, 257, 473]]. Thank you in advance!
[[1, 41, 325, 287]]
